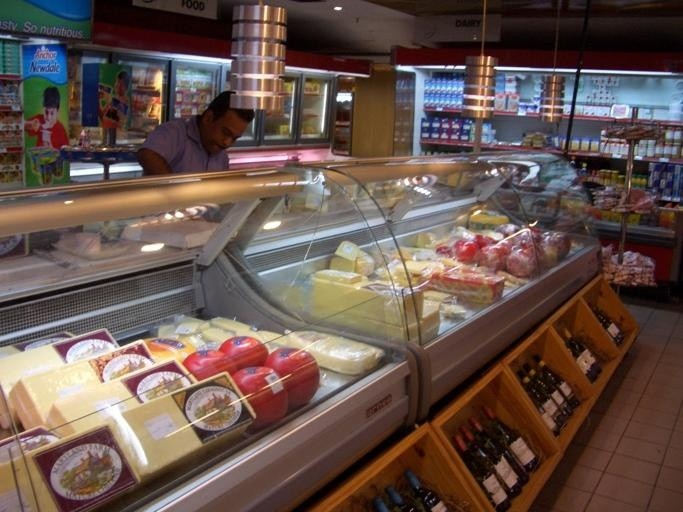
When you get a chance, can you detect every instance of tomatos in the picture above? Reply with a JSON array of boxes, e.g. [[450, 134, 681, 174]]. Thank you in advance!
[[436, 223, 571, 279]]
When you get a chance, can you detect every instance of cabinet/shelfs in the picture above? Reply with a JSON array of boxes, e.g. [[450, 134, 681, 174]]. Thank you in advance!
[[0, 148, 604, 511], [391, 48, 683, 304], [534, 304, 536, 307], [0, 22, 373, 184]]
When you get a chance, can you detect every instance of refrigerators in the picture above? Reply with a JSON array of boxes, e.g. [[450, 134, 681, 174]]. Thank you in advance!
[[66, 21, 373, 153]]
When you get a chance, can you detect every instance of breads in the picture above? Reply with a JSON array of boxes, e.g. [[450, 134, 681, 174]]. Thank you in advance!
[[426, 265, 505, 304], [142, 336, 198, 364]]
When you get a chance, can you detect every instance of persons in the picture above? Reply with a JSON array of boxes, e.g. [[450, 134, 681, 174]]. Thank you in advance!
[[97, 68, 131, 147], [23, 84, 68, 153], [134, 90, 256, 222]]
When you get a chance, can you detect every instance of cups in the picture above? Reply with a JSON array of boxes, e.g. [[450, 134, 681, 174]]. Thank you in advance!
[[40, 128, 53, 144]]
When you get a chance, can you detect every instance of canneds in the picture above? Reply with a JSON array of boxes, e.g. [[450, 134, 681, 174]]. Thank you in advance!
[[598, 169, 626, 188], [598, 122, 683, 159]]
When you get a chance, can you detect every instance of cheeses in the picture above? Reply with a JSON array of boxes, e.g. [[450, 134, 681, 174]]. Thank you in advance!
[[278, 231, 466, 346], [159, 316, 386, 376], [1, 327, 256, 512]]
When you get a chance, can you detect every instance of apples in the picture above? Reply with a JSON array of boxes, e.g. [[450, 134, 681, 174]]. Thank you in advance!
[[262, 348, 321, 410], [183, 349, 238, 382], [218, 337, 269, 370], [232, 366, 288, 433]]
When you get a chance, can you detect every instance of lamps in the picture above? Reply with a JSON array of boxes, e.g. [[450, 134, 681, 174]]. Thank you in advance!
[[538, 1, 565, 123], [460, 0, 498, 122], [228, 0, 289, 113]]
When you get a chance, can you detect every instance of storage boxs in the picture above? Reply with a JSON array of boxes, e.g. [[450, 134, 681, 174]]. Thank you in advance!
[[304, 421, 484, 511], [431, 363, 564, 512], [500, 272, 643, 449]]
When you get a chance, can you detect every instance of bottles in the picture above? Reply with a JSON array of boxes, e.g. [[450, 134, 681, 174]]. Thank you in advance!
[[452, 402, 542, 512], [421, 71, 473, 144], [369, 466, 453, 512], [516, 301, 625, 438]]
